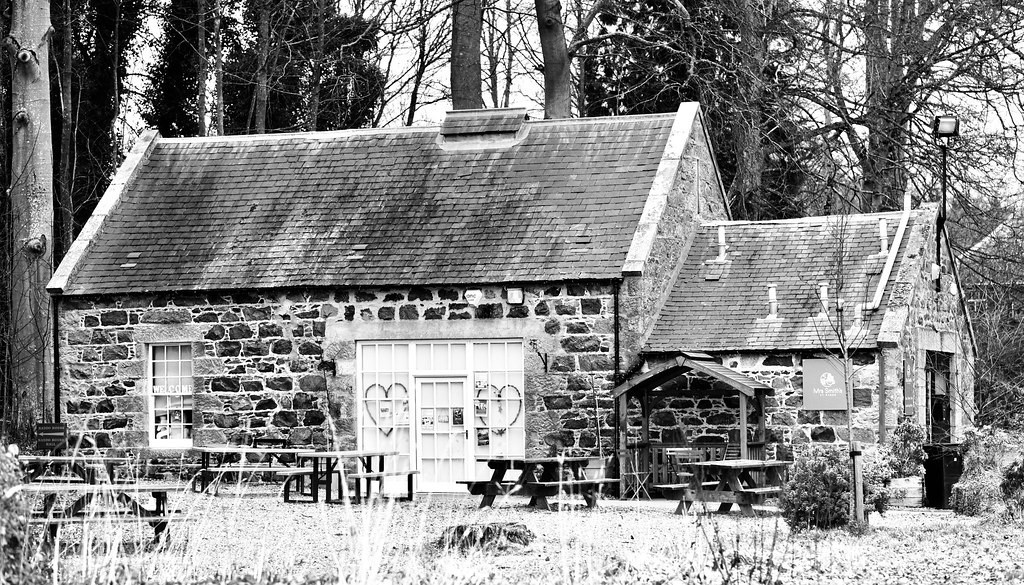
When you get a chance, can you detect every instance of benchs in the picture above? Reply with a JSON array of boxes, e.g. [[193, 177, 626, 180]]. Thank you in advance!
[[279, 467, 344, 504], [732, 484, 785, 497], [454, 477, 519, 491], [183, 459, 300, 492], [14, 448, 198, 551], [527, 474, 622, 494], [210, 466, 304, 491], [344, 470, 422, 501], [657, 482, 733, 491]]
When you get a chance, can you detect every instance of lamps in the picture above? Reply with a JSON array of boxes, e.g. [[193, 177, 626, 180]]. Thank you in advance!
[[506, 287, 526, 306]]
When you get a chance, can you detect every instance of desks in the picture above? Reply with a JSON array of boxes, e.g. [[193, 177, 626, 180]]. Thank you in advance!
[[677, 455, 798, 518], [297, 446, 399, 505], [477, 456, 596, 511], [190, 444, 317, 494]]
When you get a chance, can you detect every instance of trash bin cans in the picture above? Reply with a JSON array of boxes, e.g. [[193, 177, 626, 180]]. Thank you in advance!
[[923, 442, 963, 509]]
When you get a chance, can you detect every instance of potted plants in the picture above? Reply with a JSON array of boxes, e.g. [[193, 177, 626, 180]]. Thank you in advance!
[[879, 415, 931, 509]]
